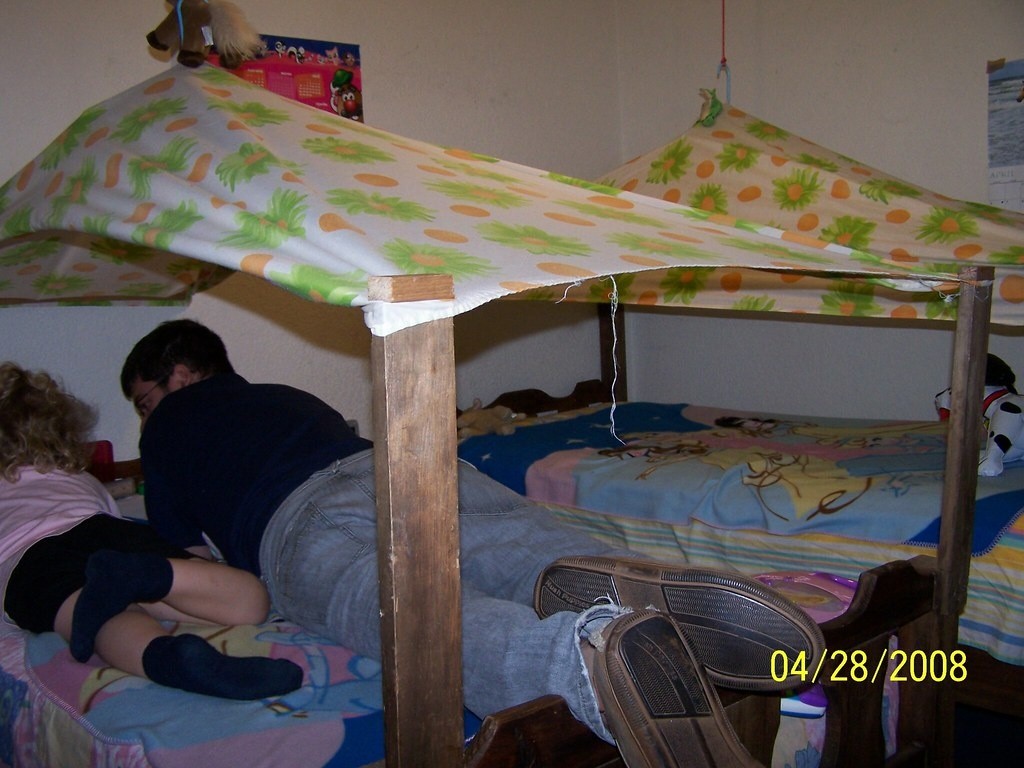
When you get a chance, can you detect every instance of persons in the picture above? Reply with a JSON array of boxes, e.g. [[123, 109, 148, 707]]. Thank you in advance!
[[0, 358, 304, 700], [120, 321, 826, 768]]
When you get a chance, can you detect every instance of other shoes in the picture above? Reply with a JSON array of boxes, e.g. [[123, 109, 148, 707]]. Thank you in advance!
[[533, 555, 827, 692], [588, 609, 765, 768]]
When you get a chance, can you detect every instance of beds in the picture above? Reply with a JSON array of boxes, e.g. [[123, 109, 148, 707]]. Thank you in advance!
[[0, 58, 993, 768], [449, 107, 1024, 719]]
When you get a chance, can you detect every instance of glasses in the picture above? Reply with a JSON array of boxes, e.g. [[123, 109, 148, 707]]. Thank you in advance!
[[134, 369, 196, 417]]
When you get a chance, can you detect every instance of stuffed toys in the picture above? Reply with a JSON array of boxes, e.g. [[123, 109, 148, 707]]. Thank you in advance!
[[935, 352, 1024, 476], [698, 88, 722, 126], [146, 0, 267, 70], [458, 398, 526, 438]]
